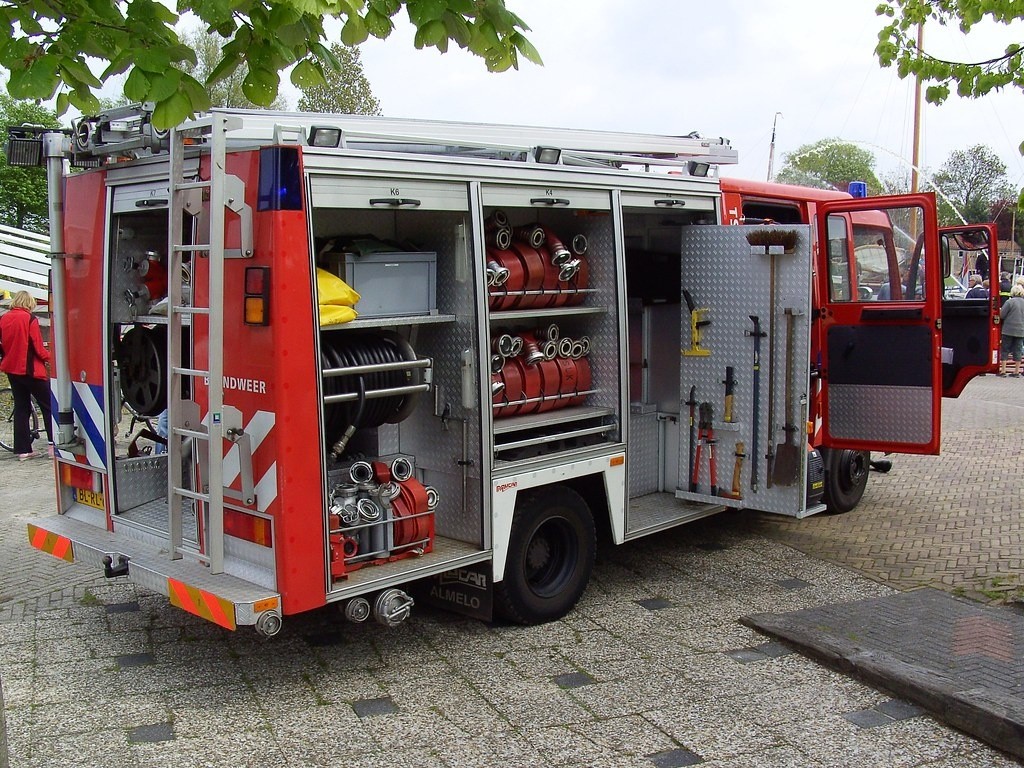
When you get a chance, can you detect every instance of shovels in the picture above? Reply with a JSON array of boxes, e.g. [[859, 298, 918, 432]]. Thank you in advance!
[[771, 307, 801, 487]]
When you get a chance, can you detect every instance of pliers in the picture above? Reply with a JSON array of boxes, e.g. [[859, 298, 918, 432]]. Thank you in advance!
[[690, 402, 717, 496]]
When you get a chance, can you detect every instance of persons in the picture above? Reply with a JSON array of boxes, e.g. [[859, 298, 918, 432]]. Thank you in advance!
[[877, 254, 925, 300], [964, 250, 1024, 378], [0, 291, 53, 461]]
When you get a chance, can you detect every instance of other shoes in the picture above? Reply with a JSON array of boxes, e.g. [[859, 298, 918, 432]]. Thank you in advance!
[[1009, 372, 1020, 378], [48, 441, 54, 460], [18, 451, 43, 461], [996, 371, 1006, 376], [979, 373, 986, 376]]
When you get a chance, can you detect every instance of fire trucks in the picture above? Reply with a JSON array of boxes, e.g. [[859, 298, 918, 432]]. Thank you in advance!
[[8, 103, 1000, 638]]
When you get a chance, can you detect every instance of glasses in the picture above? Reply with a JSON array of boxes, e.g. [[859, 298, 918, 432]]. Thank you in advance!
[[969, 280, 974, 282]]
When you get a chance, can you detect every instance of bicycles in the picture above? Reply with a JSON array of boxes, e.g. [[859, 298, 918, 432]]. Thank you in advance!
[[0, 387, 46, 452]]
